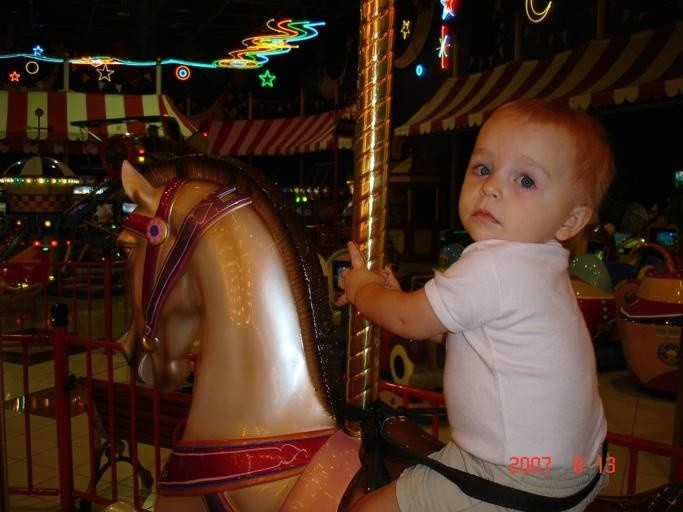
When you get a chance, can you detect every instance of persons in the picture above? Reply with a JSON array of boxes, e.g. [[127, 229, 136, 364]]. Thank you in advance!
[[332, 94, 617, 512]]
